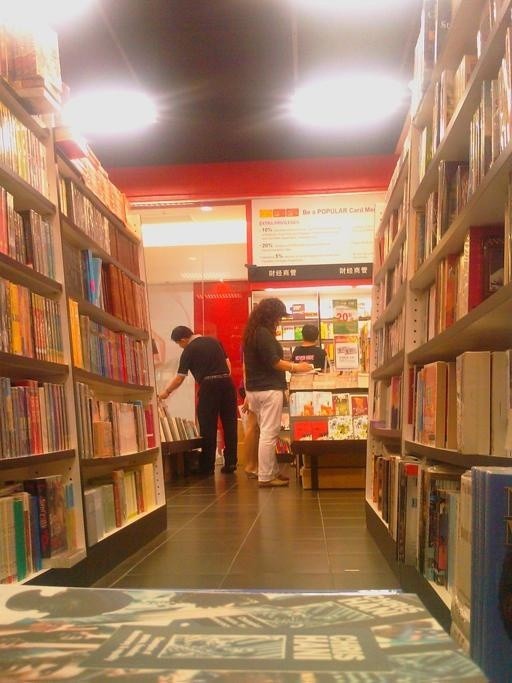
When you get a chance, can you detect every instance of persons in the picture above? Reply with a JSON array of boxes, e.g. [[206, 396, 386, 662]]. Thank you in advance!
[[159, 324, 239, 478], [235, 378, 261, 479], [235, 296, 314, 488]]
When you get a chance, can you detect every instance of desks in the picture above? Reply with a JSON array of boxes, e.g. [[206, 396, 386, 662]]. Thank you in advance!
[[162, 438, 201, 481], [290, 440, 367, 490]]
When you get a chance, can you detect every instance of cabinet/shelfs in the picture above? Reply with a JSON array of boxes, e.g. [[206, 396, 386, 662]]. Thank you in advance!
[[0, 75, 167, 588], [365, 0, 512, 635]]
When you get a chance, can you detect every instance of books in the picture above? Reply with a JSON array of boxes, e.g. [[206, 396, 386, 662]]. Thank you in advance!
[[160, 404, 201, 443], [251, 292, 369, 441], [370, 5, 511, 681], [0, 17, 159, 598]]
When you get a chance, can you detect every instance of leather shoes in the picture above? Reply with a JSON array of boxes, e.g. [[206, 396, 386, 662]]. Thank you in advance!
[[191, 468, 214, 474], [258, 477, 289, 487], [221, 464, 237, 472]]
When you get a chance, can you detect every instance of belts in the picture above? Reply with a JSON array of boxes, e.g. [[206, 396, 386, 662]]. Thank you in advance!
[[203, 372, 229, 379]]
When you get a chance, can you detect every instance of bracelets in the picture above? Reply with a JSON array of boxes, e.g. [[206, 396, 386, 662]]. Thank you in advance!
[[165, 388, 172, 396]]
